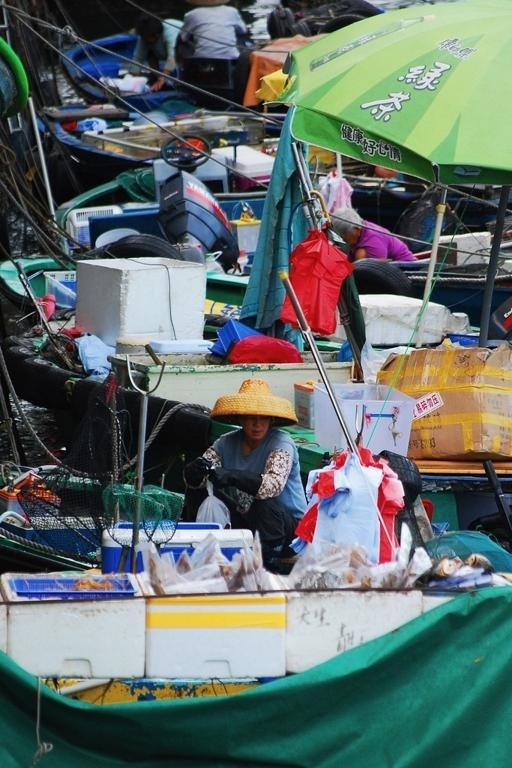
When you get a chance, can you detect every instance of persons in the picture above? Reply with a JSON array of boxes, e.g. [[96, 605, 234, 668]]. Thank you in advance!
[[180, 0, 247, 60], [178, 379, 308, 577], [130, 13, 186, 92], [331, 208, 419, 262]]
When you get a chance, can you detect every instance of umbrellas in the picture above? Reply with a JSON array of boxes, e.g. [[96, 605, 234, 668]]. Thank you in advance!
[[263, 0, 512, 349]]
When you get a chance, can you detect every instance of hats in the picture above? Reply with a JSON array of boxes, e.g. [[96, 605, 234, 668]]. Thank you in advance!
[[211, 381, 299, 427]]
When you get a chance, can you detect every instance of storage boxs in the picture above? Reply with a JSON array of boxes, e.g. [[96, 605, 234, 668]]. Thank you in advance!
[[0, 572, 423, 683], [313, 342, 512, 460], [75, 258, 207, 346]]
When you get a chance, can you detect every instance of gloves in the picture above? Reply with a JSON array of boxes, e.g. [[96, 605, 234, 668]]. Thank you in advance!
[[183, 457, 214, 486], [207, 466, 262, 495]]
